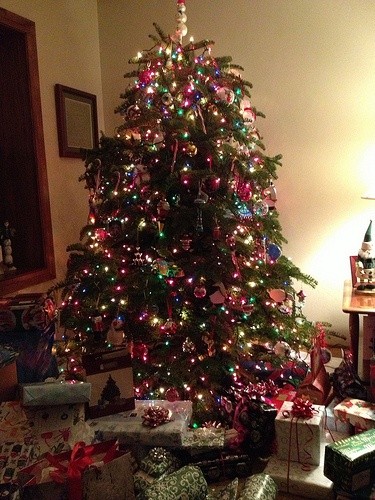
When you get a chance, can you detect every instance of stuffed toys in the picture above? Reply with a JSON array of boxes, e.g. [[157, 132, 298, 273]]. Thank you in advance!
[[239, 98, 256, 125], [106, 319, 125, 345], [355, 218, 375, 290]]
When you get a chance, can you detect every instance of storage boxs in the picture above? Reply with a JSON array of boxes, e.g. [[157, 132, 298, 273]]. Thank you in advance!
[[0, 344, 372, 500]]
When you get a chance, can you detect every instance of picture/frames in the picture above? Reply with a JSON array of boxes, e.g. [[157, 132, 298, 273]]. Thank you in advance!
[[52, 81, 104, 162]]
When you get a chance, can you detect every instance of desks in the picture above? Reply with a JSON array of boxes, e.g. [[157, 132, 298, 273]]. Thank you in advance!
[[340, 278, 375, 380]]
